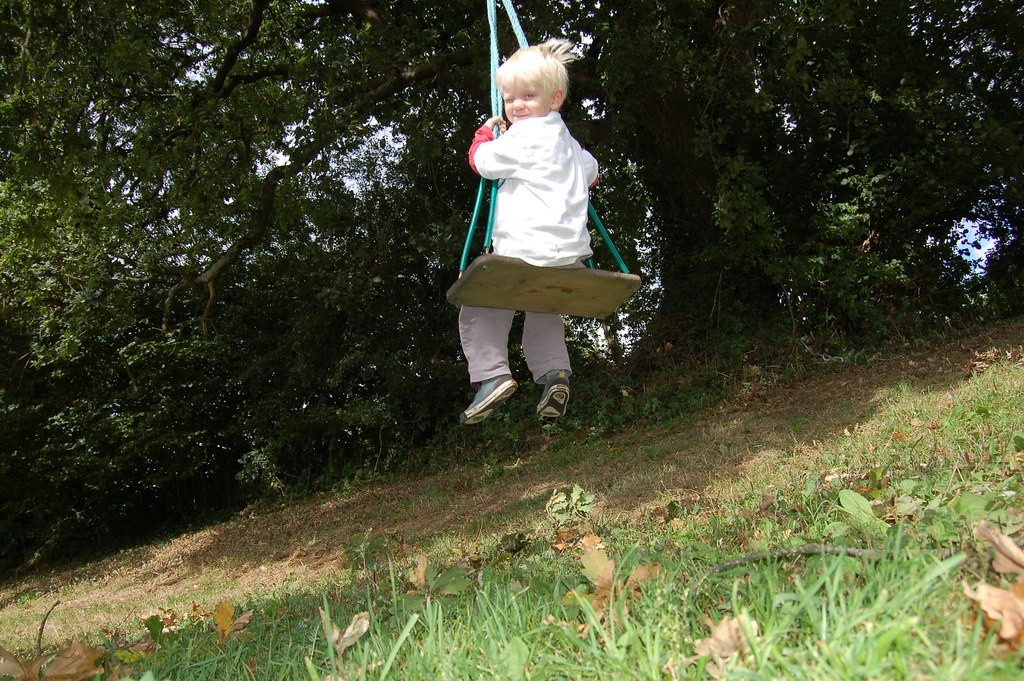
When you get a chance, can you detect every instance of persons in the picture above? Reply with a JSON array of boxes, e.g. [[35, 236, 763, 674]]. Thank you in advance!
[[457, 38, 599, 426]]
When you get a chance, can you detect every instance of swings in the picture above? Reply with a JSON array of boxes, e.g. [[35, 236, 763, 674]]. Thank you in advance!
[[444, 0, 645, 321]]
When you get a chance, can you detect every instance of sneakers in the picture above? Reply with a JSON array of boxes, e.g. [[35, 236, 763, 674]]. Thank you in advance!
[[459, 374, 518, 425], [537, 371, 570, 418]]
